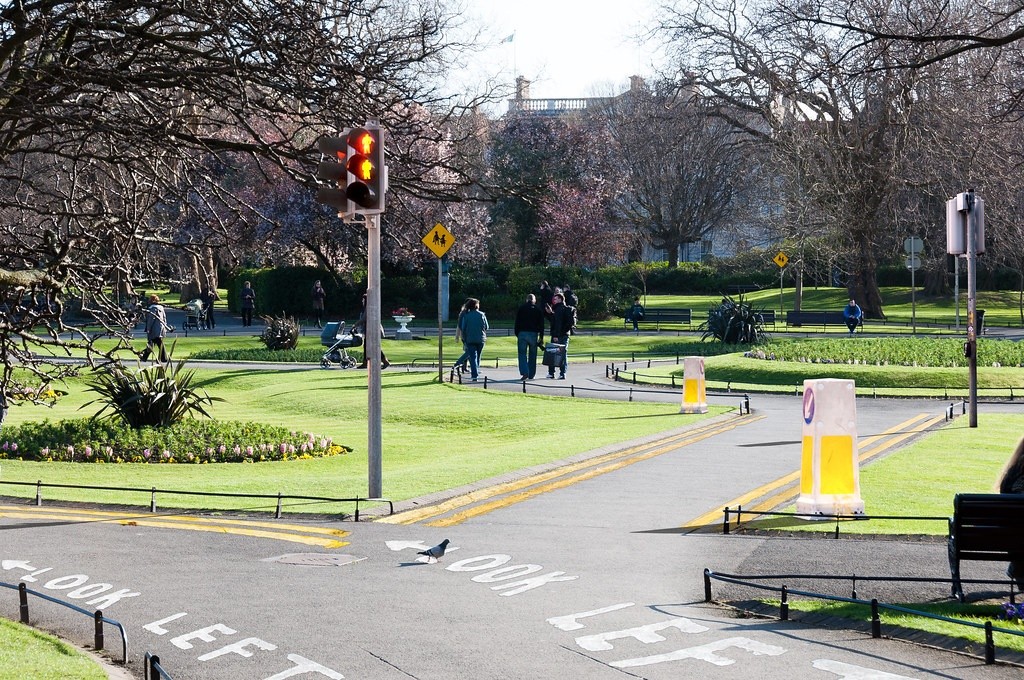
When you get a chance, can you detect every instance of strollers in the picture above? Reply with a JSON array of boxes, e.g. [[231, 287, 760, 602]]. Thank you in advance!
[[319, 320, 363, 369], [183, 299, 210, 330]]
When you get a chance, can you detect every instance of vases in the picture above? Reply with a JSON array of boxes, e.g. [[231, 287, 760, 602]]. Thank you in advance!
[[393, 315, 416, 332]]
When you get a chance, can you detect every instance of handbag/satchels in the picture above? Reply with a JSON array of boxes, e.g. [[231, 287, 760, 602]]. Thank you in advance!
[[542, 342, 566, 367]]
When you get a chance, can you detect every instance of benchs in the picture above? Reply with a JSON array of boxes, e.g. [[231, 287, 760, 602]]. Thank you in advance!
[[624, 308, 692, 332], [948, 493, 1024, 602], [786, 311, 864, 332], [749, 310, 776, 330]]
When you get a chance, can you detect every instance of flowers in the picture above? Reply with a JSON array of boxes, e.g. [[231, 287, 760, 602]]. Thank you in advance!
[[392, 307, 412, 316]]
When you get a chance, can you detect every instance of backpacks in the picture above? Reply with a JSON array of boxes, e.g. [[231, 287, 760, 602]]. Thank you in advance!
[[556, 305, 578, 332]]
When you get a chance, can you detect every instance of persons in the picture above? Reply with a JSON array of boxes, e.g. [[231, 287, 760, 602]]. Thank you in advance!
[[312, 280, 326, 329], [515, 294, 545, 380], [201, 281, 216, 329], [544, 294, 570, 380], [539, 281, 579, 335], [353, 294, 390, 370], [842, 299, 861, 334], [1000, 437, 1024, 592], [241, 281, 256, 327], [139, 294, 170, 363], [451, 298, 489, 380], [626, 297, 645, 331]]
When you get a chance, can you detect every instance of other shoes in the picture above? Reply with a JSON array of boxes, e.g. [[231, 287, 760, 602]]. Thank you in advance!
[[451, 367, 454, 371], [356, 363, 367, 369], [381, 361, 390, 370], [464, 369, 471, 374], [521, 373, 529, 380], [528, 375, 536, 380], [546, 372, 555, 378], [556, 375, 565, 379], [456, 367, 459, 373], [472, 378, 478, 382]]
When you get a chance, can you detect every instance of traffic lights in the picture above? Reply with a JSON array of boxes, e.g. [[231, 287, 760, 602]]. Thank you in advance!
[[347, 126, 385, 215], [317, 125, 350, 218]]
